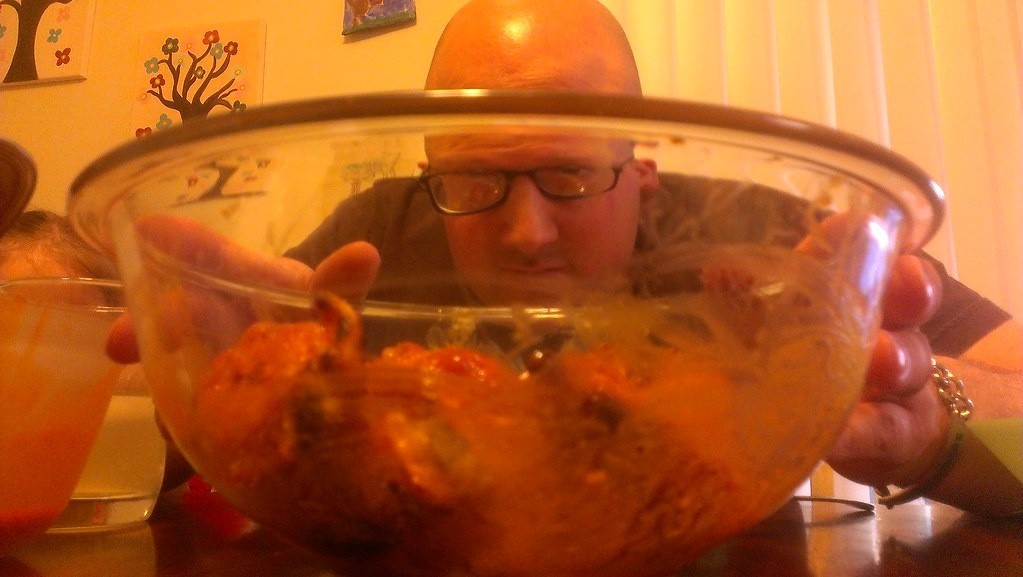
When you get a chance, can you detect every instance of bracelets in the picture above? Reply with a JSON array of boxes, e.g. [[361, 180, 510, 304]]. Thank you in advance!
[[873, 356, 974, 509]]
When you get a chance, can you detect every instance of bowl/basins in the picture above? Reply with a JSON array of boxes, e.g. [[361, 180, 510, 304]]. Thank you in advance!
[[65, 89, 947, 577], [0, 296, 126, 561]]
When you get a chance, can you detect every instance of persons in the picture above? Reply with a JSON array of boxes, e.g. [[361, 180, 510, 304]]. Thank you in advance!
[[1, 211, 153, 393], [105, 0, 1023, 577]]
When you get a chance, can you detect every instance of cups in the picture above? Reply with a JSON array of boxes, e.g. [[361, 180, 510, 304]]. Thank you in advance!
[[0, 278, 167, 536]]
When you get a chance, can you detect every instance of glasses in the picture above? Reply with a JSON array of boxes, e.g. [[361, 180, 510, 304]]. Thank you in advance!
[[417, 155, 635, 217]]
